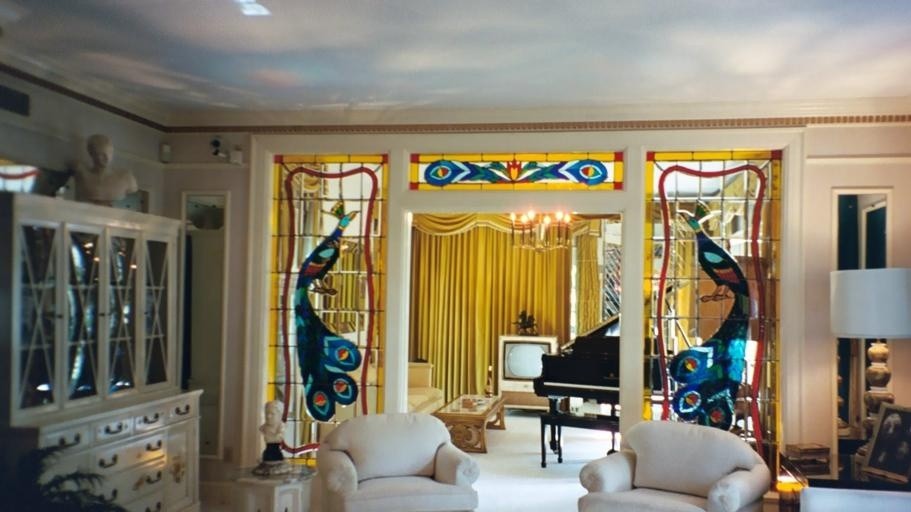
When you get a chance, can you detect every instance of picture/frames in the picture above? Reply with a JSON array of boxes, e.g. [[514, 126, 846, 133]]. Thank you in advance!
[[866, 400, 910, 483]]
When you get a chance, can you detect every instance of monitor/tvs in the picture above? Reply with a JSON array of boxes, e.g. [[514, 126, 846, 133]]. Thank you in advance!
[[498, 335, 559, 393]]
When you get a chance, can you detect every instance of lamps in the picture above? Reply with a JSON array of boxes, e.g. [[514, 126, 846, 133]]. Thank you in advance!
[[504, 211, 573, 252], [826, 185, 892, 447], [830, 267, 911, 460]]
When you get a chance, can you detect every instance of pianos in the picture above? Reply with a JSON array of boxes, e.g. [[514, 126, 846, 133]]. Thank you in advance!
[[535, 285, 674, 467]]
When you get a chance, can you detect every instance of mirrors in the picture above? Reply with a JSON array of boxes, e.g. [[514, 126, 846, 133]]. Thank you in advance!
[[178, 188, 229, 466]]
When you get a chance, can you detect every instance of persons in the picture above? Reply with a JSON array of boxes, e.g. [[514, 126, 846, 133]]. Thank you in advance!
[[73, 134, 138, 201], [258, 400, 285, 444]]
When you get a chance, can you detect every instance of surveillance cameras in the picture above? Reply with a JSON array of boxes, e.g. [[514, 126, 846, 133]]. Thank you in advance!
[[212, 135, 221, 148]]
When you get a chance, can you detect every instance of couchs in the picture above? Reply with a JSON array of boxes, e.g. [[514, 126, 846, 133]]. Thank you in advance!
[[574, 423, 768, 510], [408, 359, 448, 411], [317, 411, 483, 512]]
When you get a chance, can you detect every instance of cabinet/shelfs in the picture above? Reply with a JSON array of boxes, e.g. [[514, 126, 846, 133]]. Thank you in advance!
[[230, 465, 319, 512], [1, 189, 189, 419], [18, 391, 202, 512], [779, 446, 910, 511]]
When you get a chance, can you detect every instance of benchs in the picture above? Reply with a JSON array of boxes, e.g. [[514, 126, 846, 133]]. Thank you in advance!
[[532, 408, 621, 466]]
